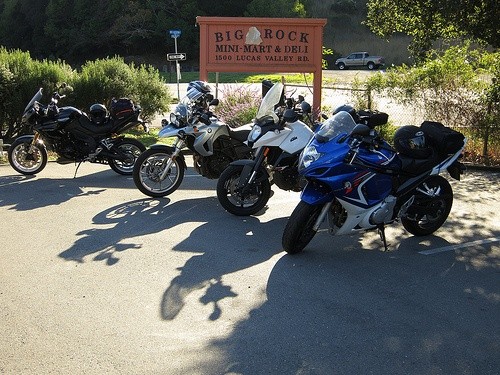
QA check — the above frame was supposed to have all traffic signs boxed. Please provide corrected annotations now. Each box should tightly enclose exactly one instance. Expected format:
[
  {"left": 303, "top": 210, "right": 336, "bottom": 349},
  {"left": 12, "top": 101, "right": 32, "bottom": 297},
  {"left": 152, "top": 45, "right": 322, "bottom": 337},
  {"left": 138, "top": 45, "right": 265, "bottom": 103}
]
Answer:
[{"left": 167, "top": 52, "right": 187, "bottom": 61}]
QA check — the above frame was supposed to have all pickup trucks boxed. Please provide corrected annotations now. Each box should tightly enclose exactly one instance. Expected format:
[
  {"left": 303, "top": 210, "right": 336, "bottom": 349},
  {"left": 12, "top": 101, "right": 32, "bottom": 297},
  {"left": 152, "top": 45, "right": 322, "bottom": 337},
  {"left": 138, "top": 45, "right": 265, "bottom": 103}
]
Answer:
[{"left": 335, "top": 51, "right": 384, "bottom": 70}]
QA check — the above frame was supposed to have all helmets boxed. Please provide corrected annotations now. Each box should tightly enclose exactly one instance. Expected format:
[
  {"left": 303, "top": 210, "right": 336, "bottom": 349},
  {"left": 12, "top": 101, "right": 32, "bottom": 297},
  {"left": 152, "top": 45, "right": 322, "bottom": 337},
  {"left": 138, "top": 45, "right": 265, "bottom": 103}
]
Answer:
[
  {"left": 395, "top": 125, "right": 428, "bottom": 158},
  {"left": 187, "top": 81, "right": 211, "bottom": 101},
  {"left": 90, "top": 104, "right": 107, "bottom": 120},
  {"left": 333, "top": 105, "right": 356, "bottom": 122}
]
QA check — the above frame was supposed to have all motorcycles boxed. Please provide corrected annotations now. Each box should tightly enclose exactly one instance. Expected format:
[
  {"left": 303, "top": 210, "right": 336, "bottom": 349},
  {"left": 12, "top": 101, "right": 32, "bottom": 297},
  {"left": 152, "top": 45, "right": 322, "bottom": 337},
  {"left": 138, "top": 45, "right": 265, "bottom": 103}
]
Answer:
[
  {"left": 8, "top": 82, "right": 149, "bottom": 175},
  {"left": 132, "top": 80, "right": 468, "bottom": 255}
]
